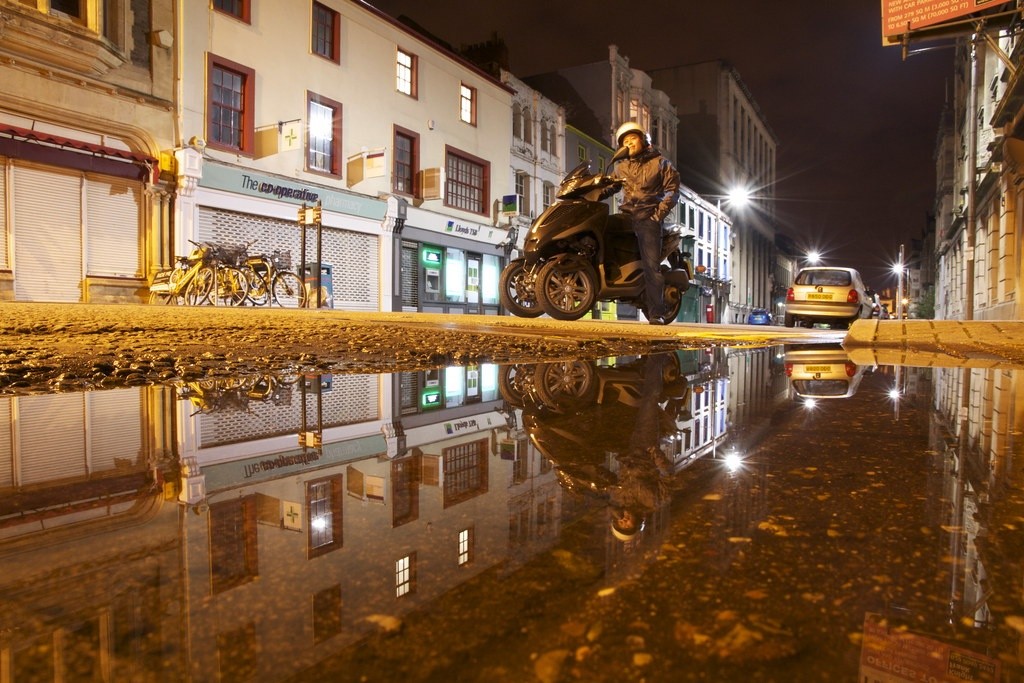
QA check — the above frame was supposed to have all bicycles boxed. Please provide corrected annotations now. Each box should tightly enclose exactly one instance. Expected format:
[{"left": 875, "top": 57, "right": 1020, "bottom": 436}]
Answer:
[{"left": 148, "top": 238, "right": 307, "bottom": 310}]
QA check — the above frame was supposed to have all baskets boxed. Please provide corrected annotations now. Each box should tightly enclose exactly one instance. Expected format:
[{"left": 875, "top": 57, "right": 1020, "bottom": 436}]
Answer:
[
  {"left": 229, "top": 249, "right": 248, "bottom": 266},
  {"left": 271, "top": 251, "right": 291, "bottom": 268}
]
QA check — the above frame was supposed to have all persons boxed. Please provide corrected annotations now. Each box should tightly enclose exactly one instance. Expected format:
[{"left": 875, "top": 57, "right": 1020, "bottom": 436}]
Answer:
[
  {"left": 578, "top": 443, "right": 680, "bottom": 549},
  {"left": 601, "top": 121, "right": 681, "bottom": 324}
]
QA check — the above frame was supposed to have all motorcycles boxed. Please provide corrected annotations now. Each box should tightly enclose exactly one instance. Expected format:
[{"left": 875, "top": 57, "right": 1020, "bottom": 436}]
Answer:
[
  {"left": 498, "top": 353, "right": 714, "bottom": 501},
  {"left": 500, "top": 146, "right": 706, "bottom": 327}
]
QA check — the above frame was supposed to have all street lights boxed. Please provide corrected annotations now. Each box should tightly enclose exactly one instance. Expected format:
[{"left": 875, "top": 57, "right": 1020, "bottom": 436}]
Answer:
[{"left": 893, "top": 245, "right": 905, "bottom": 319}]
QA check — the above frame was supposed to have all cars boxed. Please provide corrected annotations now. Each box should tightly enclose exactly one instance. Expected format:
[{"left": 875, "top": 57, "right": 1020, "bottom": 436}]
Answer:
[
  {"left": 785, "top": 267, "right": 890, "bottom": 330},
  {"left": 748, "top": 308, "right": 772, "bottom": 325},
  {"left": 783, "top": 343, "right": 871, "bottom": 400}
]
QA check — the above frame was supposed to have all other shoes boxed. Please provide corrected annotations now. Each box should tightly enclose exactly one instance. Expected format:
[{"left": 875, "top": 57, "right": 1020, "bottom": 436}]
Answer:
[
  {"left": 649, "top": 309, "right": 665, "bottom": 325},
  {"left": 649, "top": 353, "right": 665, "bottom": 363},
  {"left": 606, "top": 272, "right": 622, "bottom": 283}
]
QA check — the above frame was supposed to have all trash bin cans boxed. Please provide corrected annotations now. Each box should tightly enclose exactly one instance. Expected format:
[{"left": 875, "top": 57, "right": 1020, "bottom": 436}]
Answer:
[
  {"left": 706, "top": 304, "right": 713, "bottom": 323},
  {"left": 296, "top": 262, "right": 334, "bottom": 309}
]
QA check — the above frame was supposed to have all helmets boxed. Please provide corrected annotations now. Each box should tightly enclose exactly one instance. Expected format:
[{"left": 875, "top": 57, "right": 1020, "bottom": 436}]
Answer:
[
  {"left": 616, "top": 122, "right": 651, "bottom": 146},
  {"left": 611, "top": 520, "right": 645, "bottom": 541}
]
